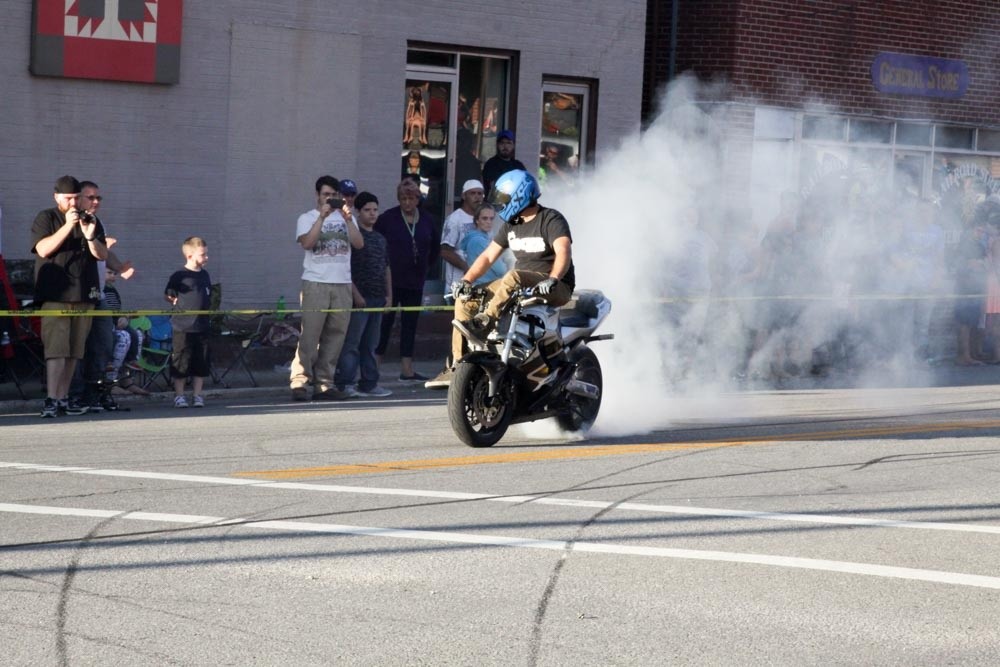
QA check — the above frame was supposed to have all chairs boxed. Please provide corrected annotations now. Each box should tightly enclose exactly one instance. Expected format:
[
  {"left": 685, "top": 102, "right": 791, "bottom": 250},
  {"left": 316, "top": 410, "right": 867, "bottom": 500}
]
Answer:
[
  {"left": 212, "top": 311, "right": 272, "bottom": 388},
  {"left": 125, "top": 335, "right": 176, "bottom": 394}
]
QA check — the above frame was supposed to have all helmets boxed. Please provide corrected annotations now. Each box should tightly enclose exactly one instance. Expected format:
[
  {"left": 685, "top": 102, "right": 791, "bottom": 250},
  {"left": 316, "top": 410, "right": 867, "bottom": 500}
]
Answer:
[{"left": 493, "top": 169, "right": 542, "bottom": 224}]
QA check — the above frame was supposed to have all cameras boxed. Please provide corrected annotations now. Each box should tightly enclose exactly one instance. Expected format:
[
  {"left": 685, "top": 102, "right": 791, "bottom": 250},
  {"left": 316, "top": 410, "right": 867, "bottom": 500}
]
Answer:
[{"left": 75, "top": 209, "right": 93, "bottom": 224}]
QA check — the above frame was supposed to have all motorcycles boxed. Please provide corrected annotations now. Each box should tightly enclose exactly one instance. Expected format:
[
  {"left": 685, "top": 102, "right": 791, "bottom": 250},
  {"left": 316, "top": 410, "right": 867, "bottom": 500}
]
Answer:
[{"left": 443, "top": 288, "right": 614, "bottom": 447}]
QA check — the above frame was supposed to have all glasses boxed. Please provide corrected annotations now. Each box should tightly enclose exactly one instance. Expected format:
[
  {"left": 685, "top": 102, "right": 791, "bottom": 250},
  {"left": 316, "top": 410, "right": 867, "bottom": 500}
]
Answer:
[{"left": 80, "top": 196, "right": 102, "bottom": 201}]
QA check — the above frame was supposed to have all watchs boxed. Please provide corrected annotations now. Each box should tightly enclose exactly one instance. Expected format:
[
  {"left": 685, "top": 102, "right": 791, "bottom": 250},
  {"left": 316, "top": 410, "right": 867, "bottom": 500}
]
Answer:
[{"left": 344, "top": 218, "right": 352, "bottom": 223}]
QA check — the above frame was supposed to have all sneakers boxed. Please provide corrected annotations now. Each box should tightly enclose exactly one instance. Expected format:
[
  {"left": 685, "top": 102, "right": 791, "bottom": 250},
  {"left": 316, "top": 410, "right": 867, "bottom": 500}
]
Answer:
[
  {"left": 424, "top": 367, "right": 456, "bottom": 390},
  {"left": 58, "top": 399, "right": 90, "bottom": 417},
  {"left": 332, "top": 384, "right": 360, "bottom": 397},
  {"left": 312, "top": 389, "right": 348, "bottom": 400},
  {"left": 41, "top": 398, "right": 58, "bottom": 419},
  {"left": 451, "top": 318, "right": 490, "bottom": 350},
  {"left": 293, "top": 388, "right": 307, "bottom": 400},
  {"left": 174, "top": 394, "right": 189, "bottom": 407},
  {"left": 356, "top": 384, "right": 392, "bottom": 397},
  {"left": 192, "top": 394, "right": 204, "bottom": 407}
]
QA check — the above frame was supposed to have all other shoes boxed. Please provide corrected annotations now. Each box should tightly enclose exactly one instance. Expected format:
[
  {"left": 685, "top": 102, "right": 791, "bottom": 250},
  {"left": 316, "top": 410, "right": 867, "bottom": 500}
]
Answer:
[
  {"left": 78, "top": 398, "right": 104, "bottom": 412},
  {"left": 398, "top": 372, "right": 429, "bottom": 383},
  {"left": 125, "top": 359, "right": 143, "bottom": 371},
  {"left": 122, "top": 383, "right": 149, "bottom": 395},
  {"left": 111, "top": 385, "right": 133, "bottom": 395},
  {"left": 101, "top": 400, "right": 119, "bottom": 410},
  {"left": 105, "top": 361, "right": 114, "bottom": 372}
]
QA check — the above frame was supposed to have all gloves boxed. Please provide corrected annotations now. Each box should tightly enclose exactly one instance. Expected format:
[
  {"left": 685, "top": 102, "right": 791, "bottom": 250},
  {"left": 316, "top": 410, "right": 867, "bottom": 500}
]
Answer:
[
  {"left": 451, "top": 280, "right": 472, "bottom": 299},
  {"left": 530, "top": 277, "right": 558, "bottom": 296}
]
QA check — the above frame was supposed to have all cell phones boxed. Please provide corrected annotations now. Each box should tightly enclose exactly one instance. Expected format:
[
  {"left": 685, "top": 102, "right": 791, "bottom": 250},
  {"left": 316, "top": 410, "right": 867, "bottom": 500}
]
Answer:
[{"left": 326, "top": 199, "right": 347, "bottom": 209}]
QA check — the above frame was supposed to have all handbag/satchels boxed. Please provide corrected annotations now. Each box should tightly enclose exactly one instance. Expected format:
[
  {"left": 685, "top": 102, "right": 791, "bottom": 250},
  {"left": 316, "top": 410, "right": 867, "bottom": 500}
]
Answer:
[{"left": 261, "top": 321, "right": 300, "bottom": 349}]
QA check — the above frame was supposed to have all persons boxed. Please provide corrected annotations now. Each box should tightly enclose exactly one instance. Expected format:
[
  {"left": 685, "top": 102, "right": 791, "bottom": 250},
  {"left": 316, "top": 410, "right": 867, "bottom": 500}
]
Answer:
[
  {"left": 540, "top": 145, "right": 578, "bottom": 183},
  {"left": 482, "top": 130, "right": 527, "bottom": 200},
  {"left": 425, "top": 169, "right": 575, "bottom": 389},
  {"left": 440, "top": 180, "right": 507, "bottom": 306},
  {"left": 672, "top": 194, "right": 1000, "bottom": 390},
  {"left": 164, "top": 237, "right": 211, "bottom": 408},
  {"left": 31, "top": 175, "right": 143, "bottom": 418},
  {"left": 290, "top": 175, "right": 434, "bottom": 400}
]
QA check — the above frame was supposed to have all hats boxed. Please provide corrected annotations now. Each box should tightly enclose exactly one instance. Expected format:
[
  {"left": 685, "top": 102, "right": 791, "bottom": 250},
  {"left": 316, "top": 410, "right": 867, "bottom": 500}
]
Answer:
[
  {"left": 338, "top": 180, "right": 358, "bottom": 195},
  {"left": 54, "top": 176, "right": 81, "bottom": 193},
  {"left": 462, "top": 180, "right": 484, "bottom": 193},
  {"left": 497, "top": 130, "right": 514, "bottom": 141}
]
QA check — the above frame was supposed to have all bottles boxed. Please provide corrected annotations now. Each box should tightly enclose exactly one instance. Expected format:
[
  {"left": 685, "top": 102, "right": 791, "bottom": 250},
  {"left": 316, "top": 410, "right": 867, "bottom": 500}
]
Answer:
[
  {"left": 424, "top": 294, "right": 431, "bottom": 312},
  {"left": 2, "top": 332, "right": 9, "bottom": 344},
  {"left": 277, "top": 296, "right": 287, "bottom": 319}
]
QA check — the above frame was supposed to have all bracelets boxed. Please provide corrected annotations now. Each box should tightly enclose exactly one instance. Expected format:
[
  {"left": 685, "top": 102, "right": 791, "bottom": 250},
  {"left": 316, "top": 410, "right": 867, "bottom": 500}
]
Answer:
[{"left": 86, "top": 236, "right": 97, "bottom": 241}]
